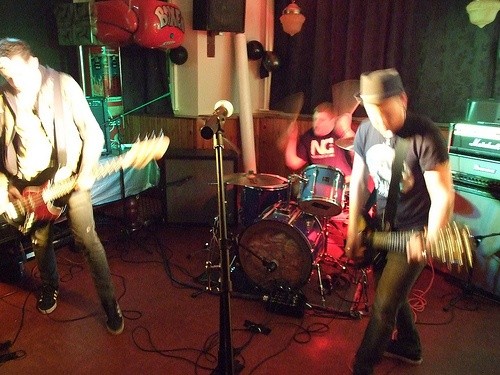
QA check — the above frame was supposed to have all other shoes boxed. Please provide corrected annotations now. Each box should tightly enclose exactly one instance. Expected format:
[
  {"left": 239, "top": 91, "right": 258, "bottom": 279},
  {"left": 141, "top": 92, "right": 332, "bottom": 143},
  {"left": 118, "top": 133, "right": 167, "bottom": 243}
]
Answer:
[{"left": 384, "top": 339, "right": 422, "bottom": 364}]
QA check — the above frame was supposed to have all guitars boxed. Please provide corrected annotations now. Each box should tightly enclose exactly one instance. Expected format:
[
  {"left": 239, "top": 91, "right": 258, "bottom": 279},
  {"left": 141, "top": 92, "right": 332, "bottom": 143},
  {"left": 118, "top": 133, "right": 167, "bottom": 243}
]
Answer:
[
  {"left": 347, "top": 211, "right": 479, "bottom": 276},
  {"left": 1, "top": 128, "right": 171, "bottom": 235}
]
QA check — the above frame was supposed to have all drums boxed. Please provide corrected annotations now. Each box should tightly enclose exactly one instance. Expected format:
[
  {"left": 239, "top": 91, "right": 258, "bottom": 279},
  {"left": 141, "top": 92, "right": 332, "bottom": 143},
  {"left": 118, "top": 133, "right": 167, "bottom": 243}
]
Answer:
[
  {"left": 236, "top": 200, "right": 324, "bottom": 292},
  {"left": 242, "top": 184, "right": 292, "bottom": 225},
  {"left": 314, "top": 215, "right": 330, "bottom": 253},
  {"left": 297, "top": 163, "right": 345, "bottom": 217}
]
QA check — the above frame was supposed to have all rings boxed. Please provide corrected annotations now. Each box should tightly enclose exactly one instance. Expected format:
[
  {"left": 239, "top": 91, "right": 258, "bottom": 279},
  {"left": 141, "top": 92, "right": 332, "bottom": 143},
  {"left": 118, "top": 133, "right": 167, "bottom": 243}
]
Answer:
[{"left": 347, "top": 247, "right": 354, "bottom": 251}]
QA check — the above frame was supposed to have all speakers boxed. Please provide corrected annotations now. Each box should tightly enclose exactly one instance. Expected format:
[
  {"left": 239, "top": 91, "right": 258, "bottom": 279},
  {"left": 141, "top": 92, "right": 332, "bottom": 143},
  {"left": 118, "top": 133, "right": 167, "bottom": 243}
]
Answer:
[
  {"left": 425, "top": 182, "right": 500, "bottom": 302},
  {"left": 192, "top": 0, "right": 246, "bottom": 33}
]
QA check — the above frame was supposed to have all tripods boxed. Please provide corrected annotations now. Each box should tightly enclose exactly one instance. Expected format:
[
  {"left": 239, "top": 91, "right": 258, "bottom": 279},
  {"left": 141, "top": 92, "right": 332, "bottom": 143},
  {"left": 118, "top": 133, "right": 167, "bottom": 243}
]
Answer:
[{"left": 97, "top": 169, "right": 153, "bottom": 254}]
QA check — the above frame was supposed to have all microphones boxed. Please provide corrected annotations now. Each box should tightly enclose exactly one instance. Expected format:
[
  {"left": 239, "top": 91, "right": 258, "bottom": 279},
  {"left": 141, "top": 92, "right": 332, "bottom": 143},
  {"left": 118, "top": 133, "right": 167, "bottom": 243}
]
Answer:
[{"left": 201, "top": 100, "right": 233, "bottom": 140}]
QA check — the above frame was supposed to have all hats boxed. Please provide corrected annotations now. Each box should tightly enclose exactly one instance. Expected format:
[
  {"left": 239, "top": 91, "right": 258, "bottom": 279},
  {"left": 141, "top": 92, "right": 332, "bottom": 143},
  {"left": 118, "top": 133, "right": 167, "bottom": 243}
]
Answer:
[{"left": 354, "top": 68, "right": 403, "bottom": 100}]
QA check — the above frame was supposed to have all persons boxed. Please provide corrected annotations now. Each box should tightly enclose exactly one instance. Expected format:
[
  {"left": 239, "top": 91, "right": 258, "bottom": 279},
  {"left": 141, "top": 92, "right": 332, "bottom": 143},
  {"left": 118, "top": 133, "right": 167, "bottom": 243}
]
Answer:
[
  {"left": 346, "top": 67, "right": 455, "bottom": 375},
  {"left": 284, "top": 102, "right": 354, "bottom": 176},
  {"left": 0, "top": 37, "right": 124, "bottom": 334}
]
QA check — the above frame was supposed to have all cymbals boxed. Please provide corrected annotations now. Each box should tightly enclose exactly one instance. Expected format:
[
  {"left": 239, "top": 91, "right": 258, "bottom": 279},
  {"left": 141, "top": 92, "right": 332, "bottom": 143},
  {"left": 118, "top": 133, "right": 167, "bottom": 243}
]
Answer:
[
  {"left": 335, "top": 136, "right": 355, "bottom": 153},
  {"left": 224, "top": 173, "right": 290, "bottom": 187}
]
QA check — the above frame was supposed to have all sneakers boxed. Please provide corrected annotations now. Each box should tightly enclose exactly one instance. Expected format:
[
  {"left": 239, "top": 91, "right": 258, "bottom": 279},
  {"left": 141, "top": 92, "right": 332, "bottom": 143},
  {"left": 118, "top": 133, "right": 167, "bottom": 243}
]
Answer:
[
  {"left": 105, "top": 302, "right": 124, "bottom": 335},
  {"left": 38, "top": 286, "right": 59, "bottom": 315}
]
